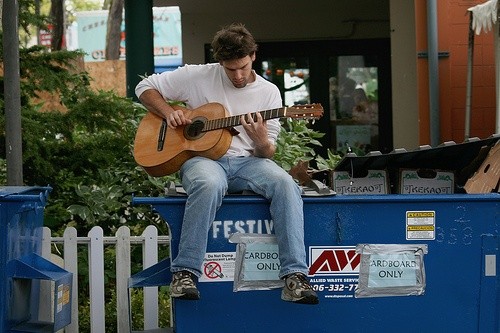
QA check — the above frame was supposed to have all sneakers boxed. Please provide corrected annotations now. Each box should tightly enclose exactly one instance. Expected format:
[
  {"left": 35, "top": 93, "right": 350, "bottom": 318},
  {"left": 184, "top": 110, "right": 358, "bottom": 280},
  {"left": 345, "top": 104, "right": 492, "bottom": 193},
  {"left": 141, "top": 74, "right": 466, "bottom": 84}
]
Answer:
[
  {"left": 281, "top": 272, "right": 319, "bottom": 305},
  {"left": 169, "top": 272, "right": 201, "bottom": 300}
]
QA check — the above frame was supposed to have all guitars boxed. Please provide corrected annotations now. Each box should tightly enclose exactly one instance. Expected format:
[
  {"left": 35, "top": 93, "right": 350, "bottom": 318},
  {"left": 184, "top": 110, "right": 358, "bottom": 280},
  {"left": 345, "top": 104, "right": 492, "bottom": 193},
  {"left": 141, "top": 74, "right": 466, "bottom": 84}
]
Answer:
[{"left": 132, "top": 101, "right": 325, "bottom": 179}]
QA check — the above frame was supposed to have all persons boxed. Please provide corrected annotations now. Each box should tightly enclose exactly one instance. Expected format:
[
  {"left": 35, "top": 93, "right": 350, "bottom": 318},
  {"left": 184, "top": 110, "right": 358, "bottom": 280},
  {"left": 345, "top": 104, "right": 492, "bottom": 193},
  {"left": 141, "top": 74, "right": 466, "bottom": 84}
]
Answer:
[{"left": 135, "top": 22, "right": 320, "bottom": 305}]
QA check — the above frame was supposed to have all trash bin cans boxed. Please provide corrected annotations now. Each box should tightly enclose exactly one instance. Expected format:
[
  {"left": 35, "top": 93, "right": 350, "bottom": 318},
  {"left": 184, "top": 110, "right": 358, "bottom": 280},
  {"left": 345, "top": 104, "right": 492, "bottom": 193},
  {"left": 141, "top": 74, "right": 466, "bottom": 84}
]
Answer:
[{"left": 1, "top": 185, "right": 74, "bottom": 333}]
